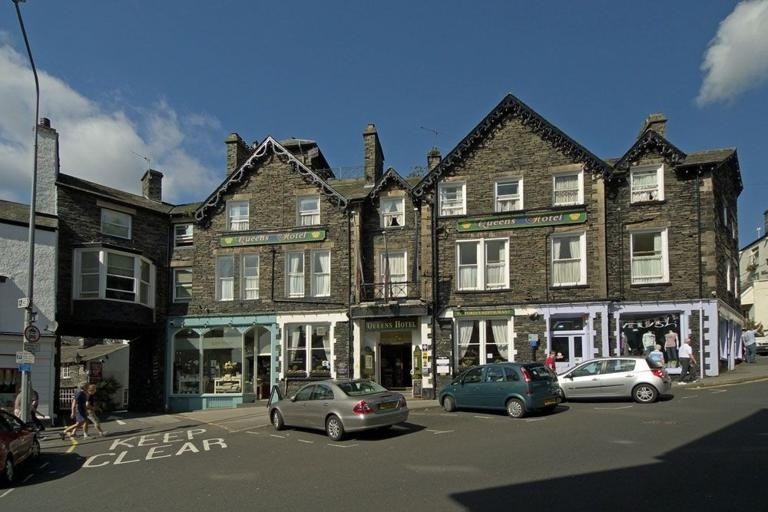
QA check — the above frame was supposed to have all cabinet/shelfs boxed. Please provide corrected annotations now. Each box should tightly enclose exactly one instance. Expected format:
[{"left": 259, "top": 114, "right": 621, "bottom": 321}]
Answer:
[{"left": 178, "top": 377, "right": 241, "bottom": 393}]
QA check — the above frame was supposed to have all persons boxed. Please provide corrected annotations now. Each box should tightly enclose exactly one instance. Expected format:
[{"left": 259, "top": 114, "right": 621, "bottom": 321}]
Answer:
[
  {"left": 542, "top": 351, "right": 556, "bottom": 373},
  {"left": 615, "top": 331, "right": 631, "bottom": 356},
  {"left": 646, "top": 344, "right": 664, "bottom": 373},
  {"left": 13, "top": 391, "right": 22, "bottom": 440},
  {"left": 641, "top": 329, "right": 656, "bottom": 357},
  {"left": 70, "top": 384, "right": 109, "bottom": 441},
  {"left": 676, "top": 337, "right": 696, "bottom": 387},
  {"left": 30, "top": 390, "right": 45, "bottom": 436},
  {"left": 742, "top": 321, "right": 763, "bottom": 364},
  {"left": 57, "top": 382, "right": 89, "bottom": 441},
  {"left": 663, "top": 329, "right": 679, "bottom": 363}
]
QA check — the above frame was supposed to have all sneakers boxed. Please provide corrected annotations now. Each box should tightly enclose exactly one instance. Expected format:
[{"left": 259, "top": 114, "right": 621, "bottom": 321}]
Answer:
[
  {"left": 59, "top": 432, "right": 66, "bottom": 440},
  {"left": 71, "top": 430, "right": 109, "bottom": 440}
]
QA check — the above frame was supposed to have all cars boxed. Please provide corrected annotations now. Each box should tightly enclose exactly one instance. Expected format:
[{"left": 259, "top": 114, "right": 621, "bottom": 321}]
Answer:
[
  {"left": 0, "top": 409, "right": 40, "bottom": 481},
  {"left": 269, "top": 380, "right": 409, "bottom": 441},
  {"left": 439, "top": 362, "right": 562, "bottom": 418},
  {"left": 556, "top": 358, "right": 670, "bottom": 403}
]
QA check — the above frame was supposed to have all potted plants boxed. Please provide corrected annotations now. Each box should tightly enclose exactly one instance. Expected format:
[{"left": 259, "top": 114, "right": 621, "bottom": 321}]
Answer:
[{"left": 223, "top": 360, "right": 238, "bottom": 377}]
